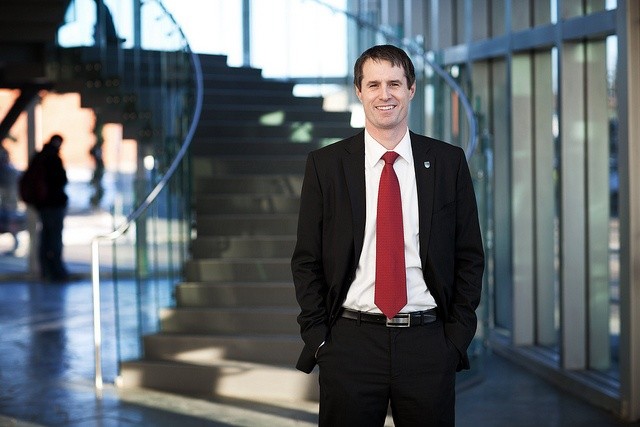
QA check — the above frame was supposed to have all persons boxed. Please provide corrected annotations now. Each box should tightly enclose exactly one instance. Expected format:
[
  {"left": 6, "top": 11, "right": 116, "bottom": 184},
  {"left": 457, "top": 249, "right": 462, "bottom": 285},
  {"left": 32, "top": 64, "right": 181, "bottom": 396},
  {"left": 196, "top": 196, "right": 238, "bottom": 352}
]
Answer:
[
  {"left": 288, "top": 46, "right": 487, "bottom": 426},
  {"left": 21, "top": 134, "right": 81, "bottom": 284}
]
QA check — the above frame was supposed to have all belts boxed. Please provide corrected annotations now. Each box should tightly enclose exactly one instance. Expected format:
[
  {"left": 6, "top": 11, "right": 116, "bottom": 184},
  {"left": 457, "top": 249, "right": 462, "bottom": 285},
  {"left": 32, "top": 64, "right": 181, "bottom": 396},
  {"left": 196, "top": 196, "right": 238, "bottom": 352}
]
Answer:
[{"left": 341, "top": 308, "right": 437, "bottom": 327}]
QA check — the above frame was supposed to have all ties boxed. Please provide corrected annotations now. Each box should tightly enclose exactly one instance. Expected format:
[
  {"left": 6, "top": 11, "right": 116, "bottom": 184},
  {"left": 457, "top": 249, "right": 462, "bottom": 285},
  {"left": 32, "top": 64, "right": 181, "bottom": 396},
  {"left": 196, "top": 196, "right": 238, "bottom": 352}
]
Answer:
[{"left": 375, "top": 152, "right": 407, "bottom": 320}]
